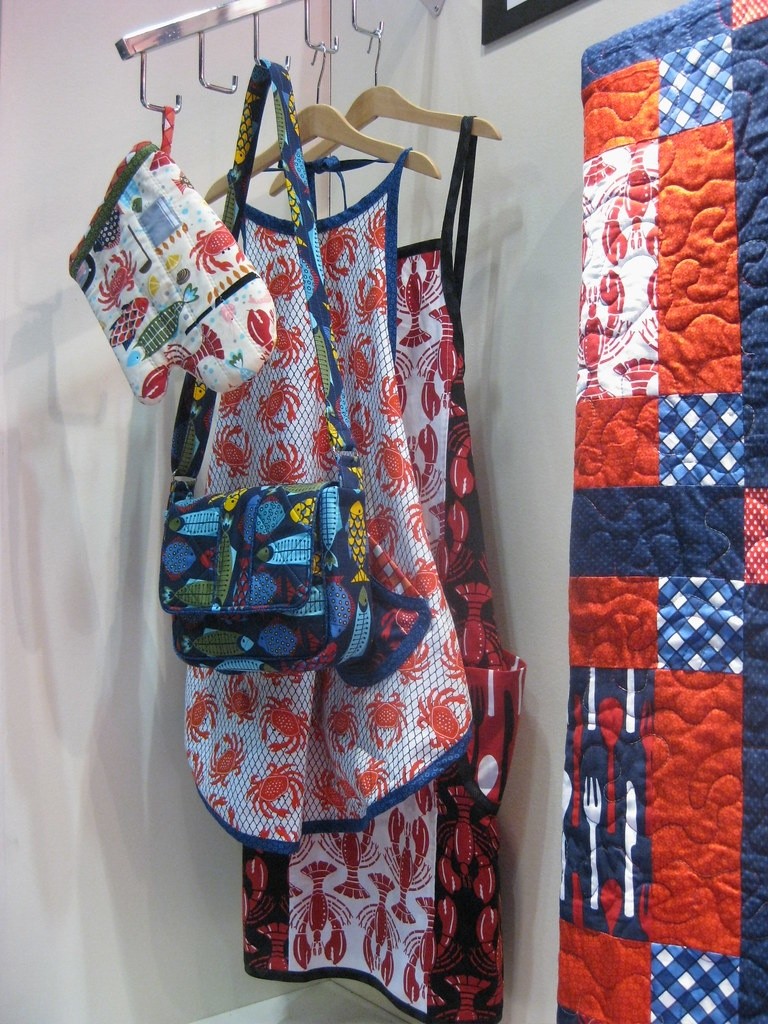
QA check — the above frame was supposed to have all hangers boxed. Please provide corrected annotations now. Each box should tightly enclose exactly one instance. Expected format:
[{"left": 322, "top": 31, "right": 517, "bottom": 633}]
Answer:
[
  {"left": 270, "top": 30, "right": 502, "bottom": 197},
  {"left": 204, "top": 41, "right": 441, "bottom": 206}
]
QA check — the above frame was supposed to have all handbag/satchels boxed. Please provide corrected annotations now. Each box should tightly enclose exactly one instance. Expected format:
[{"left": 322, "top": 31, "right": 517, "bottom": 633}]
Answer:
[
  {"left": 157, "top": 58, "right": 375, "bottom": 673},
  {"left": 68, "top": 105, "right": 277, "bottom": 406}
]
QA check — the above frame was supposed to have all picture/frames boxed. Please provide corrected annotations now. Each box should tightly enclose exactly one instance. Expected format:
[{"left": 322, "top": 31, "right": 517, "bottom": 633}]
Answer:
[{"left": 481, "top": 0, "right": 577, "bottom": 45}]
